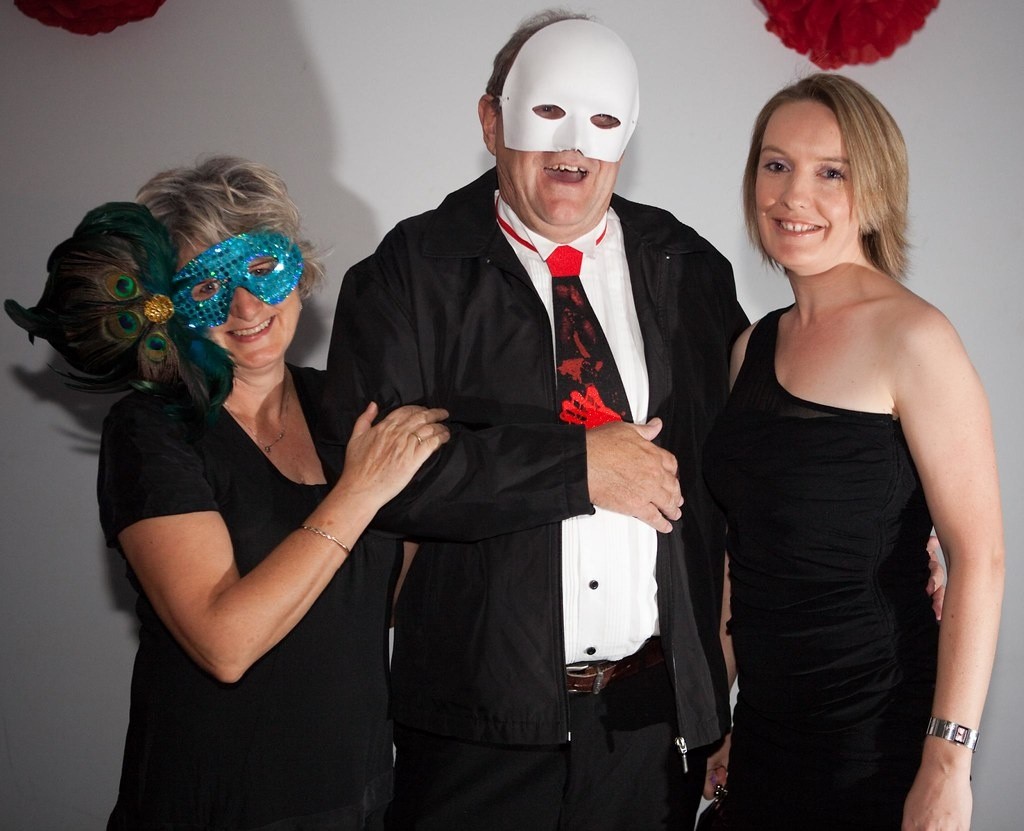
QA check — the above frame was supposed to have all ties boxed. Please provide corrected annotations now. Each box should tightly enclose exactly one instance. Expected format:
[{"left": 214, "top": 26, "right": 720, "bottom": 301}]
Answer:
[{"left": 541, "top": 245, "right": 633, "bottom": 432}]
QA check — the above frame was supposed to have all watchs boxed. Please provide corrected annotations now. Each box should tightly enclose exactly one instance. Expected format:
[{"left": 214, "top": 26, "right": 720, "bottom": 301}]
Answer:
[{"left": 923, "top": 715, "right": 981, "bottom": 754}]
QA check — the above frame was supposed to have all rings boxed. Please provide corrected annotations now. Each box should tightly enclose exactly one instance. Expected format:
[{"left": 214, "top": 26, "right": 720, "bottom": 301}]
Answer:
[{"left": 410, "top": 432, "right": 423, "bottom": 446}]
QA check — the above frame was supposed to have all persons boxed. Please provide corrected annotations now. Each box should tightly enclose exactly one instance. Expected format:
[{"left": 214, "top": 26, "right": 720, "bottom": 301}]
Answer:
[
  {"left": 319, "top": 5, "right": 951, "bottom": 830},
  {"left": 694, "top": 69, "right": 1008, "bottom": 831},
  {"left": 93, "top": 141, "right": 455, "bottom": 831}
]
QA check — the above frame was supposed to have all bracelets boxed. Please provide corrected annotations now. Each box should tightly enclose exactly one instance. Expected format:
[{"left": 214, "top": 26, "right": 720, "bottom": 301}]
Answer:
[{"left": 296, "top": 524, "right": 352, "bottom": 557}]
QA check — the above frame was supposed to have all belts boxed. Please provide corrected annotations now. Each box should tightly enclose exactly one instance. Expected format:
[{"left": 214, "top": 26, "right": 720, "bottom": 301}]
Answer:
[{"left": 562, "top": 649, "right": 669, "bottom": 695}]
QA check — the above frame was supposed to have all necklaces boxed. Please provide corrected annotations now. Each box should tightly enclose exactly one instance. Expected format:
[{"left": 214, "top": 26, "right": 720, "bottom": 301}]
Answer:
[{"left": 222, "top": 370, "right": 294, "bottom": 454}]
